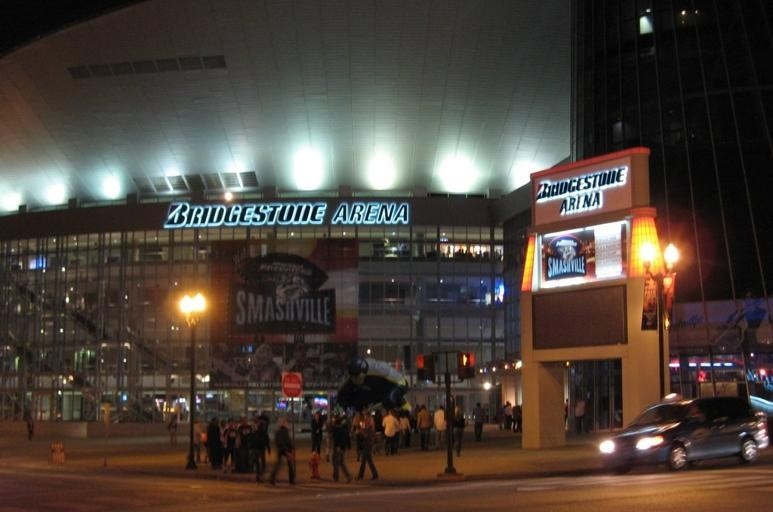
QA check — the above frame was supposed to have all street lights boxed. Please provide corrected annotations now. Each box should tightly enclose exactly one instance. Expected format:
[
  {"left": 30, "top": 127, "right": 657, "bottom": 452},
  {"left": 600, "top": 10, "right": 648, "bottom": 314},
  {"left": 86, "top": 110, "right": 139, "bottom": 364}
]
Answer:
[
  {"left": 638, "top": 241, "right": 679, "bottom": 402},
  {"left": 178, "top": 293, "right": 212, "bottom": 472}
]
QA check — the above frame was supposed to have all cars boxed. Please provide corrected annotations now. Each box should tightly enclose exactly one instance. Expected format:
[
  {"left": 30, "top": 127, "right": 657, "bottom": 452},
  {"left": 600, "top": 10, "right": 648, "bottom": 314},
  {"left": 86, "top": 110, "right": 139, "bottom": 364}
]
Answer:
[{"left": 596, "top": 396, "right": 771, "bottom": 473}]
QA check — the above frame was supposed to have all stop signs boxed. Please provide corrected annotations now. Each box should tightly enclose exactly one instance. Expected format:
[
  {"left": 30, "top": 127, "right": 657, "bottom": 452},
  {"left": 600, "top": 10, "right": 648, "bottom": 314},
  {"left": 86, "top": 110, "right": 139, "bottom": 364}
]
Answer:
[{"left": 281, "top": 372, "right": 304, "bottom": 398}]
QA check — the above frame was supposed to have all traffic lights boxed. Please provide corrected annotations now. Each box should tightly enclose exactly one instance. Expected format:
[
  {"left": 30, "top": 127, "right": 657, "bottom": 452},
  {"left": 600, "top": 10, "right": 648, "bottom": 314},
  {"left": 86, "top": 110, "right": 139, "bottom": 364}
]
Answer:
[
  {"left": 416, "top": 354, "right": 434, "bottom": 382},
  {"left": 458, "top": 352, "right": 476, "bottom": 379}
]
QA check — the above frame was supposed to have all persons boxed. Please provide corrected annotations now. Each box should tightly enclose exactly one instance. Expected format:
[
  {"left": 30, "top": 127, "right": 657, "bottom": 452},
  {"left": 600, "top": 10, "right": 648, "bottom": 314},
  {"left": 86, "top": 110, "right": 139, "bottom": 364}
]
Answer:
[
  {"left": 575, "top": 398, "right": 587, "bottom": 433},
  {"left": 166, "top": 415, "right": 179, "bottom": 447},
  {"left": 25, "top": 414, "right": 35, "bottom": 440},
  {"left": 190, "top": 399, "right": 524, "bottom": 486},
  {"left": 563, "top": 398, "right": 570, "bottom": 425}
]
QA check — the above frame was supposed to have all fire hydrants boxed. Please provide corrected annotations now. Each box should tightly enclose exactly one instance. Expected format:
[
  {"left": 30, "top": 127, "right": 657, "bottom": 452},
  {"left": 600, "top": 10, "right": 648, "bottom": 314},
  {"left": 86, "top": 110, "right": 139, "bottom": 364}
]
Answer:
[{"left": 308, "top": 451, "right": 322, "bottom": 479}]
